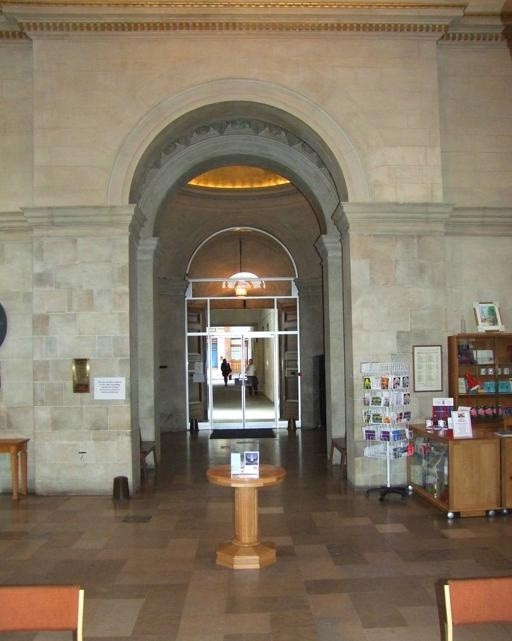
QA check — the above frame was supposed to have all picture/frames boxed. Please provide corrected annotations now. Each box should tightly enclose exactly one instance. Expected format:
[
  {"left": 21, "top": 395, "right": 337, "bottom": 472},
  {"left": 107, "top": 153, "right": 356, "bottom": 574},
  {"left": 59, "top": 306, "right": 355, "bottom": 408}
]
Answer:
[{"left": 472, "top": 299, "right": 507, "bottom": 334}]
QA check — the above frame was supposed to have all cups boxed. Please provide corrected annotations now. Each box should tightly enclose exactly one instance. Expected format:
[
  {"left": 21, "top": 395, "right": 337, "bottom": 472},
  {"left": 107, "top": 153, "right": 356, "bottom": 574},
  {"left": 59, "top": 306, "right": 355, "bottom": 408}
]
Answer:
[
  {"left": 426, "top": 420, "right": 433, "bottom": 427},
  {"left": 438, "top": 420, "right": 446, "bottom": 428},
  {"left": 480, "top": 368, "right": 509, "bottom": 375}
]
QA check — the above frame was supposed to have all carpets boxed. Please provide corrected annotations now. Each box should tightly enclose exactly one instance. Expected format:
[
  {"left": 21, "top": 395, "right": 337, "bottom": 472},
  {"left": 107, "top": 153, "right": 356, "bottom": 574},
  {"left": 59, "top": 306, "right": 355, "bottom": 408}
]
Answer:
[{"left": 210, "top": 428, "right": 276, "bottom": 439}]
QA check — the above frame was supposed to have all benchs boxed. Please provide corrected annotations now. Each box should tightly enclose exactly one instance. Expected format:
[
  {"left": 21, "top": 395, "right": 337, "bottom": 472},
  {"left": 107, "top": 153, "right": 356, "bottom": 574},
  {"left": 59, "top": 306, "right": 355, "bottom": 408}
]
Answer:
[
  {"left": 139, "top": 424, "right": 158, "bottom": 481},
  {"left": 330, "top": 437, "right": 347, "bottom": 471}
]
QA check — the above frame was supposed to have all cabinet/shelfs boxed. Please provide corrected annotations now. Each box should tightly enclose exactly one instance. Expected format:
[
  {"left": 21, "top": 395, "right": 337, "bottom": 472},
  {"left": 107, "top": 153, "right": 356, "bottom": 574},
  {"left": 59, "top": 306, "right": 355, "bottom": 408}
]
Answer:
[{"left": 405, "top": 333, "right": 512, "bottom": 520}]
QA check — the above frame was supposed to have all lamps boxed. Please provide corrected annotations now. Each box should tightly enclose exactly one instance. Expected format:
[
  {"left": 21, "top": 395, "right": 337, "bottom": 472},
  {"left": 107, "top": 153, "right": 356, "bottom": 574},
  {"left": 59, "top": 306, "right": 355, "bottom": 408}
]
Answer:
[{"left": 233, "top": 234, "right": 248, "bottom": 298}]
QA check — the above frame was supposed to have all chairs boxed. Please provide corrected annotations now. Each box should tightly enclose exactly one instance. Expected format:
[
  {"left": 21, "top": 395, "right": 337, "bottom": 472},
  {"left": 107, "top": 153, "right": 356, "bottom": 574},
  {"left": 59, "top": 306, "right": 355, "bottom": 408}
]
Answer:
[
  {"left": 0, "top": 586, "right": 84, "bottom": 641},
  {"left": 0, "top": 437, "right": 30, "bottom": 502},
  {"left": 438, "top": 578, "right": 511, "bottom": 640}
]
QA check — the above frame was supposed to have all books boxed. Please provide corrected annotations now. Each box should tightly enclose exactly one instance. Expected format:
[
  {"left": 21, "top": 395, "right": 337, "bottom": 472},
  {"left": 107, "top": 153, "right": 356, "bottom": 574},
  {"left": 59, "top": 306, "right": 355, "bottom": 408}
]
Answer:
[
  {"left": 362, "top": 373, "right": 415, "bottom": 458},
  {"left": 494, "top": 430, "right": 511, "bottom": 438}
]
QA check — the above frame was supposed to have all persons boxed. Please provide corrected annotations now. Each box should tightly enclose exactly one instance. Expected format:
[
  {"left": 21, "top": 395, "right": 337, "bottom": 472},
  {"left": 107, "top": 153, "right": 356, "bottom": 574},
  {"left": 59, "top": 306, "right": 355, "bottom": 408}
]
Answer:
[
  {"left": 220, "top": 359, "right": 232, "bottom": 388},
  {"left": 245, "top": 359, "right": 260, "bottom": 399}
]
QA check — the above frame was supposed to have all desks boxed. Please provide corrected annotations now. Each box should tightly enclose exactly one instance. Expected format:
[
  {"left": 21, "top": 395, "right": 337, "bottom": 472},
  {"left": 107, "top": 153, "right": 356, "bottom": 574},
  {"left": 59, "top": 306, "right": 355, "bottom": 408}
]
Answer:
[{"left": 205, "top": 463, "right": 285, "bottom": 571}]
